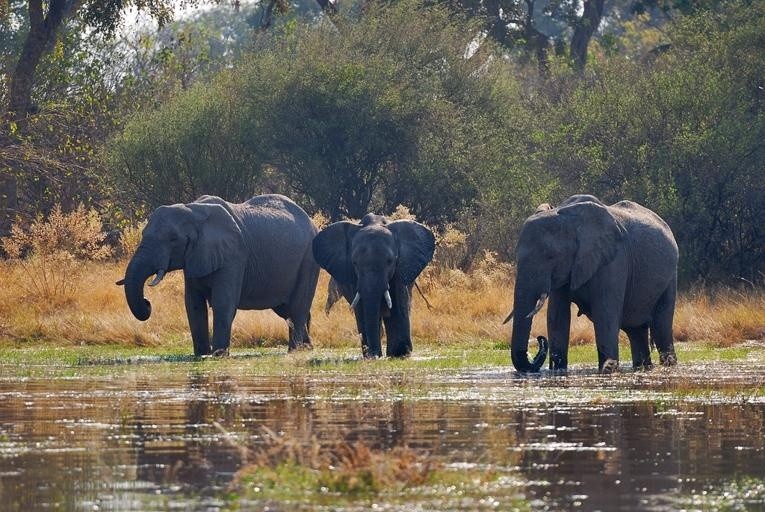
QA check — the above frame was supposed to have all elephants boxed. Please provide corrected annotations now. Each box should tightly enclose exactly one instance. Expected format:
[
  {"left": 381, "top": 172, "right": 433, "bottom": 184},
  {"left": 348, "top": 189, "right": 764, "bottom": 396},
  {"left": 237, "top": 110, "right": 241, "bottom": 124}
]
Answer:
[
  {"left": 502, "top": 193, "right": 679, "bottom": 374},
  {"left": 312, "top": 212, "right": 436, "bottom": 359},
  {"left": 116, "top": 194, "right": 322, "bottom": 358}
]
